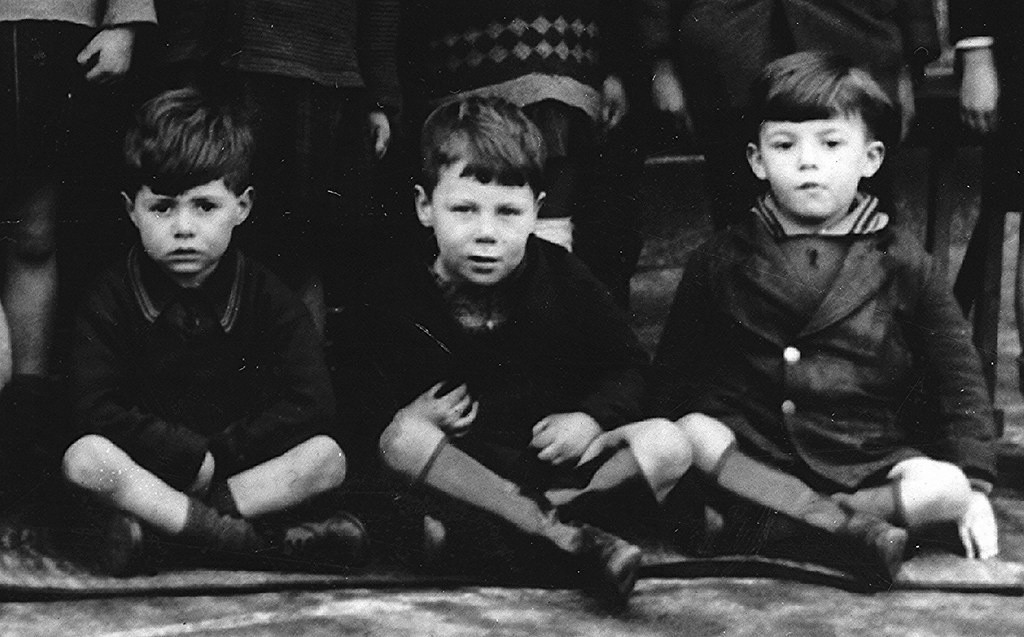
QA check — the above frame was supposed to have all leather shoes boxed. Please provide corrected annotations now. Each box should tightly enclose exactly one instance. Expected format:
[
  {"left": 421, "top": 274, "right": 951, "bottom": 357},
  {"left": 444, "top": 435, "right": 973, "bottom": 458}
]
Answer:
[
  {"left": 254, "top": 513, "right": 371, "bottom": 574},
  {"left": 836, "top": 512, "right": 908, "bottom": 579},
  {"left": 670, "top": 502, "right": 750, "bottom": 556},
  {"left": 366, "top": 495, "right": 451, "bottom": 566},
  {"left": 578, "top": 525, "right": 643, "bottom": 607},
  {"left": 102, "top": 512, "right": 153, "bottom": 577}
]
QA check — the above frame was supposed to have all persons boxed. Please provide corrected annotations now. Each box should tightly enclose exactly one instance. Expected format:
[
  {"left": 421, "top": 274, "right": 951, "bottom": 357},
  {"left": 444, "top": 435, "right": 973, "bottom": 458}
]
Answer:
[
  {"left": 326, "top": 95, "right": 693, "bottom": 602},
  {"left": 38, "top": 85, "right": 369, "bottom": 577},
  {"left": 653, "top": 51, "right": 1000, "bottom": 581},
  {"left": 1, "top": 0, "right": 1023, "bottom": 440}
]
